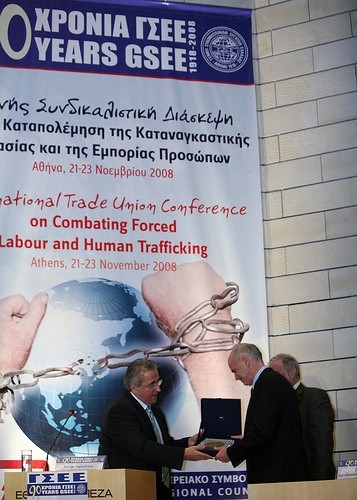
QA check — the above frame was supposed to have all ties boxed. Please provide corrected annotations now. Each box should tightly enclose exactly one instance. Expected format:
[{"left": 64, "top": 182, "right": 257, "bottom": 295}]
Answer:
[
  {"left": 145, "top": 406, "right": 170, "bottom": 489},
  {"left": 250, "top": 388, "right": 254, "bottom": 395}
]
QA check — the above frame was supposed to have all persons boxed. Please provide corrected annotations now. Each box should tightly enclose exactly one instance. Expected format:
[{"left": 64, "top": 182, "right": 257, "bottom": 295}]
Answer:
[
  {"left": 268, "top": 354, "right": 337, "bottom": 482},
  {"left": 0, "top": 260, "right": 252, "bottom": 437},
  {"left": 213, "top": 343, "right": 306, "bottom": 485},
  {"left": 98, "top": 358, "right": 214, "bottom": 500}
]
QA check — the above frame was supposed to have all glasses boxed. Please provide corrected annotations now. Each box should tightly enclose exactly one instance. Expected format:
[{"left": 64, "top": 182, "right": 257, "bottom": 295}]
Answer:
[{"left": 141, "top": 379, "right": 163, "bottom": 389}]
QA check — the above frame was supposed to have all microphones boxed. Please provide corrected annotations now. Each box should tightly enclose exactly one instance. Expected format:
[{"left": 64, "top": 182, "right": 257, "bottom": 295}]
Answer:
[{"left": 45, "top": 410, "right": 74, "bottom": 471}]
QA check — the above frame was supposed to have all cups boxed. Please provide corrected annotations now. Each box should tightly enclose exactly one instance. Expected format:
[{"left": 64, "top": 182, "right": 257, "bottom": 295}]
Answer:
[{"left": 22, "top": 450, "right": 32, "bottom": 472}]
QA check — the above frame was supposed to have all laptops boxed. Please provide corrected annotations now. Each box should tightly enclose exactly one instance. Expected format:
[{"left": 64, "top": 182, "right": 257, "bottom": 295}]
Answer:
[{"left": 192, "top": 397, "right": 242, "bottom": 445}]
